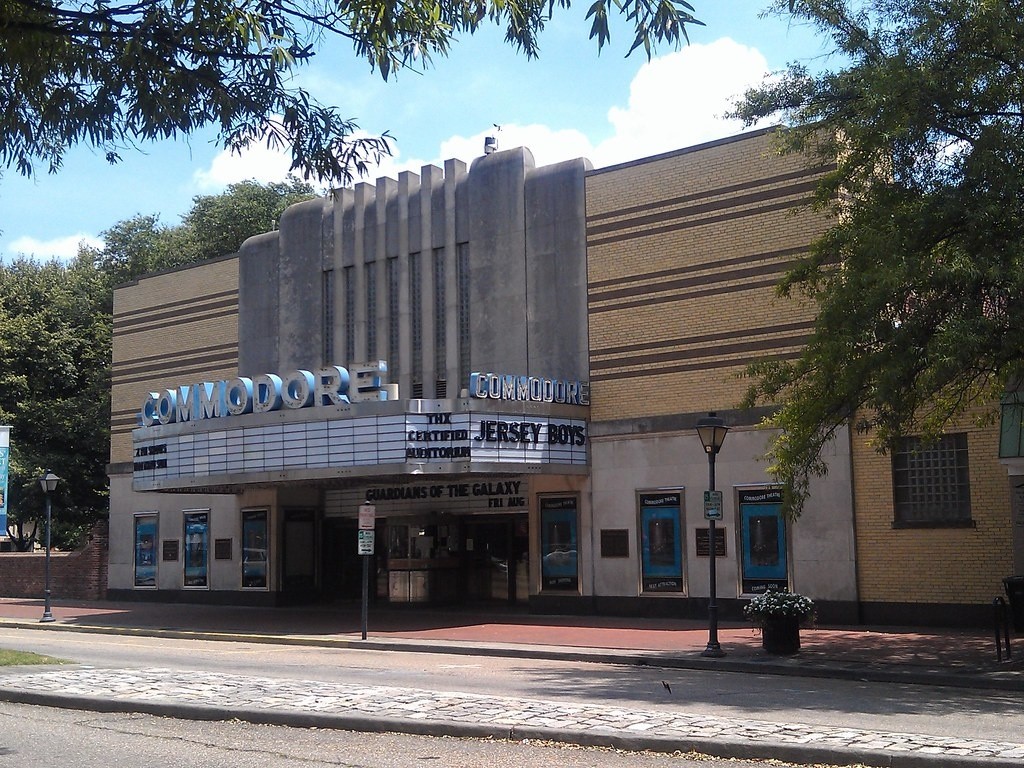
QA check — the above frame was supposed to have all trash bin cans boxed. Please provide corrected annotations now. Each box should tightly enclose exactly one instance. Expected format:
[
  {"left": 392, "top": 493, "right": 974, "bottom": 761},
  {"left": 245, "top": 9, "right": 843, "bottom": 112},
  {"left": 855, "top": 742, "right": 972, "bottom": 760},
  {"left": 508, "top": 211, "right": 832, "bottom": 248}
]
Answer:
[{"left": 1001, "top": 574, "right": 1024, "bottom": 634}]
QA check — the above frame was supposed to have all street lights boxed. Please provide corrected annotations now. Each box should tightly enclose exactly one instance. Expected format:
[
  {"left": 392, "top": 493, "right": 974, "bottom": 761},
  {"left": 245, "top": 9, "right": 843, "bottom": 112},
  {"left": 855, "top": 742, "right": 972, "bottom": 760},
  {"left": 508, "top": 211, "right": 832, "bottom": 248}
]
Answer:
[
  {"left": 695, "top": 408, "right": 735, "bottom": 654},
  {"left": 39, "top": 468, "right": 60, "bottom": 625}
]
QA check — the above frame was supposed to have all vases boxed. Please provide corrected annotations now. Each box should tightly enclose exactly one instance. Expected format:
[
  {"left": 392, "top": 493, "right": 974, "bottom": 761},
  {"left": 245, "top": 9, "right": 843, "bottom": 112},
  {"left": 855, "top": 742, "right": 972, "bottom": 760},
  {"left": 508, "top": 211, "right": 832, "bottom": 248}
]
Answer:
[{"left": 761, "top": 615, "right": 802, "bottom": 655}]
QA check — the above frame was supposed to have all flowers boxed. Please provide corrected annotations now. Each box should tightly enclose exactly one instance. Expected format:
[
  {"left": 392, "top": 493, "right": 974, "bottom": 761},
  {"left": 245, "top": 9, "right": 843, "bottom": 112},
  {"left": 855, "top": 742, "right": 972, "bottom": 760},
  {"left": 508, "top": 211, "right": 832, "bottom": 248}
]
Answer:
[{"left": 742, "top": 586, "right": 818, "bottom": 636}]
